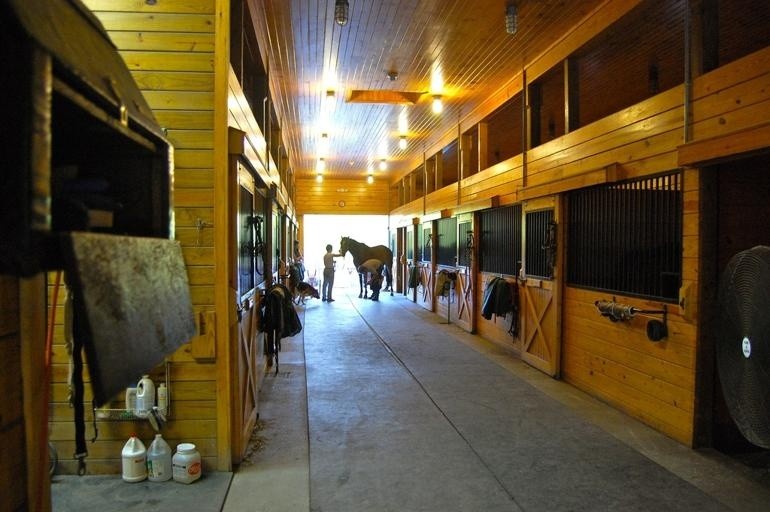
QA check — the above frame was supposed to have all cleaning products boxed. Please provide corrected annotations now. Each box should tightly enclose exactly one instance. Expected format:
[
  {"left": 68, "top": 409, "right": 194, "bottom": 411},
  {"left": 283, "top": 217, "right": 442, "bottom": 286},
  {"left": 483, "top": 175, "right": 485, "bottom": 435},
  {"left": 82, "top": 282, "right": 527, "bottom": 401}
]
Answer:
[{"left": 157, "top": 382, "right": 167, "bottom": 415}]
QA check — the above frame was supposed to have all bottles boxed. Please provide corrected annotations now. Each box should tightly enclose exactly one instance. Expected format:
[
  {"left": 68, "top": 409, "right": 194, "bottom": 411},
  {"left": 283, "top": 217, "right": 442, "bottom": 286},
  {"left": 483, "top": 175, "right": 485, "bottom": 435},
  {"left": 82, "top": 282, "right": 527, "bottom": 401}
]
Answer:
[{"left": 157, "top": 383, "right": 167, "bottom": 410}]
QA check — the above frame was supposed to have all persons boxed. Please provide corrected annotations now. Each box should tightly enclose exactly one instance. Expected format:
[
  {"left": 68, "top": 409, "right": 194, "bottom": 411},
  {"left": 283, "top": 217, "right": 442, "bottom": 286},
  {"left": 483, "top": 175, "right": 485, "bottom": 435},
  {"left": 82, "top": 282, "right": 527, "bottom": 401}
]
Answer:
[
  {"left": 294, "top": 240, "right": 302, "bottom": 264},
  {"left": 358, "top": 258, "right": 383, "bottom": 301},
  {"left": 322, "top": 244, "right": 341, "bottom": 302}
]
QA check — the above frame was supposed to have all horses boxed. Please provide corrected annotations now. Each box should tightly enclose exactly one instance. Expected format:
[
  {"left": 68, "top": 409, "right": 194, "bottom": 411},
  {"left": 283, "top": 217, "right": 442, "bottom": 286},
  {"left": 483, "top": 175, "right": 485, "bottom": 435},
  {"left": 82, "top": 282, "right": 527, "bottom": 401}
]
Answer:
[{"left": 339, "top": 235, "right": 394, "bottom": 298}]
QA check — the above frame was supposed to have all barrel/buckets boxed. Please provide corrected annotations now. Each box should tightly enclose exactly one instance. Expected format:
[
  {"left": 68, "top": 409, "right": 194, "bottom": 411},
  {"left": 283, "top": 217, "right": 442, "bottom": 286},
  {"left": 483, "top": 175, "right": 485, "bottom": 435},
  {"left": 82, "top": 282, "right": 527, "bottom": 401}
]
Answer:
[
  {"left": 120, "top": 431, "right": 148, "bottom": 482},
  {"left": 146, "top": 433, "right": 172, "bottom": 482},
  {"left": 135, "top": 374, "right": 155, "bottom": 419},
  {"left": 171, "top": 443, "right": 202, "bottom": 485}
]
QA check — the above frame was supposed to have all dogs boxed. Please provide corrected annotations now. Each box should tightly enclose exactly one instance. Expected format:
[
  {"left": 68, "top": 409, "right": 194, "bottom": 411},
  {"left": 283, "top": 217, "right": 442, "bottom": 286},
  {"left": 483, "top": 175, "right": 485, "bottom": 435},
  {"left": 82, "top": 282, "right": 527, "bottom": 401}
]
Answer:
[{"left": 291, "top": 281, "right": 320, "bottom": 306}]
